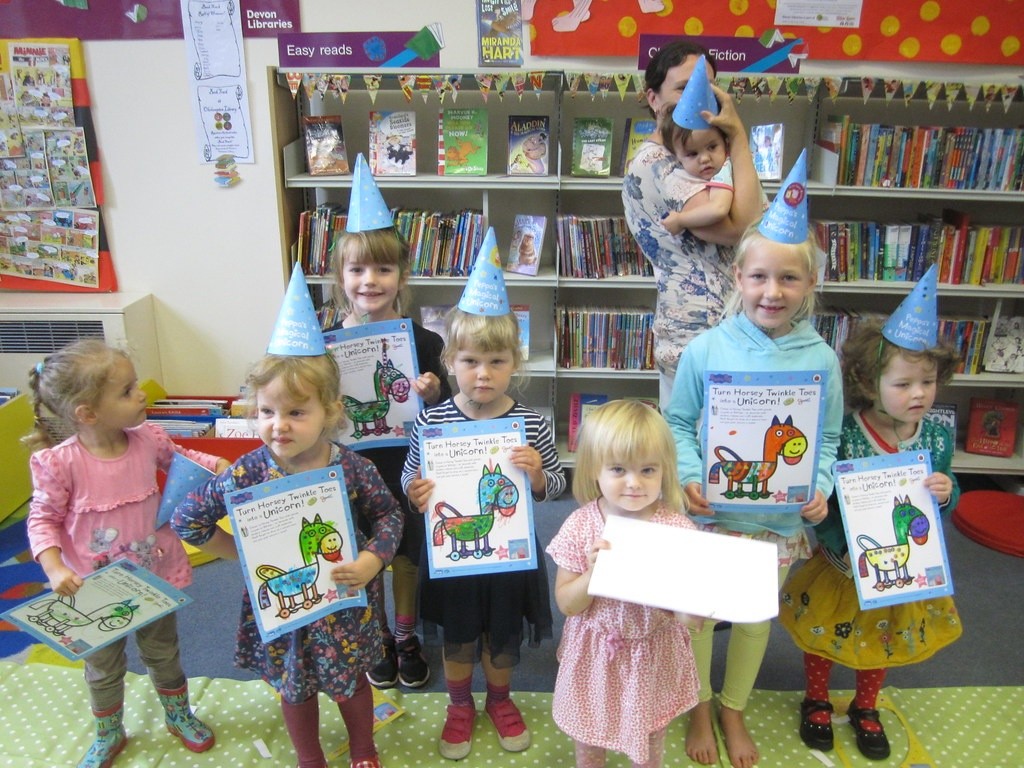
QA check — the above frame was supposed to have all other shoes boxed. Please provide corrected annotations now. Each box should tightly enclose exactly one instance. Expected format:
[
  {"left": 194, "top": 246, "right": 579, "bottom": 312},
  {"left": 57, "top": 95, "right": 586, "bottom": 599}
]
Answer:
[
  {"left": 847, "top": 704, "right": 890, "bottom": 760},
  {"left": 801, "top": 698, "right": 834, "bottom": 751}
]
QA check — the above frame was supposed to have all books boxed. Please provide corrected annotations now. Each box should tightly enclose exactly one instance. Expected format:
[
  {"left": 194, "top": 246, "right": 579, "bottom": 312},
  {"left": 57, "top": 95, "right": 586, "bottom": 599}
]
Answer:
[
  {"left": 289, "top": 114, "right": 1024, "bottom": 451},
  {"left": 142, "top": 396, "right": 266, "bottom": 440}
]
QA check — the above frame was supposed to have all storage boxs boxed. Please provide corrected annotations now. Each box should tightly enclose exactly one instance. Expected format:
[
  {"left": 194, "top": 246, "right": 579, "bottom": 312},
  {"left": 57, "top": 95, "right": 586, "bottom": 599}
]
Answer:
[{"left": 0, "top": 379, "right": 264, "bottom": 522}]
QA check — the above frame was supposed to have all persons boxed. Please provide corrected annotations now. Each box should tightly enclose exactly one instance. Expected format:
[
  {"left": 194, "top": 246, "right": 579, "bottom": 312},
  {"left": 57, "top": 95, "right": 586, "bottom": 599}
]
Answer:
[
  {"left": 659, "top": 101, "right": 772, "bottom": 236},
  {"left": 171, "top": 344, "right": 407, "bottom": 768},
  {"left": 544, "top": 396, "right": 706, "bottom": 767},
  {"left": 398, "top": 301, "right": 566, "bottom": 760},
  {"left": 24, "top": 340, "right": 231, "bottom": 768},
  {"left": 773, "top": 320, "right": 964, "bottom": 761},
  {"left": 318, "top": 226, "right": 452, "bottom": 688},
  {"left": 622, "top": 44, "right": 762, "bottom": 449},
  {"left": 664, "top": 215, "right": 845, "bottom": 767}
]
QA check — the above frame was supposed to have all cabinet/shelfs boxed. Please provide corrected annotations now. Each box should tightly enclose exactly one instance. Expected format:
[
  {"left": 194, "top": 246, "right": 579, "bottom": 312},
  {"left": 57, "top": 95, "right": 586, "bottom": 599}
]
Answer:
[{"left": 266, "top": 65, "right": 1024, "bottom": 475}]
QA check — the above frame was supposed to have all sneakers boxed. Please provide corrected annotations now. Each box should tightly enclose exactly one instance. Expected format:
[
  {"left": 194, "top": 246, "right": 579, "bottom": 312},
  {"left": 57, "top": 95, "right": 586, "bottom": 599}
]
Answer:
[
  {"left": 396, "top": 634, "right": 430, "bottom": 687},
  {"left": 484, "top": 698, "right": 530, "bottom": 751},
  {"left": 366, "top": 632, "right": 399, "bottom": 687},
  {"left": 439, "top": 704, "right": 477, "bottom": 759}
]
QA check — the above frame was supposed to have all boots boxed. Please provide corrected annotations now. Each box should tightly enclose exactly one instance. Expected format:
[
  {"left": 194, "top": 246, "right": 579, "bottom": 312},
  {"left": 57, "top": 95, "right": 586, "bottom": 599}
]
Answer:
[
  {"left": 156, "top": 678, "right": 214, "bottom": 752},
  {"left": 76, "top": 701, "right": 126, "bottom": 768}
]
